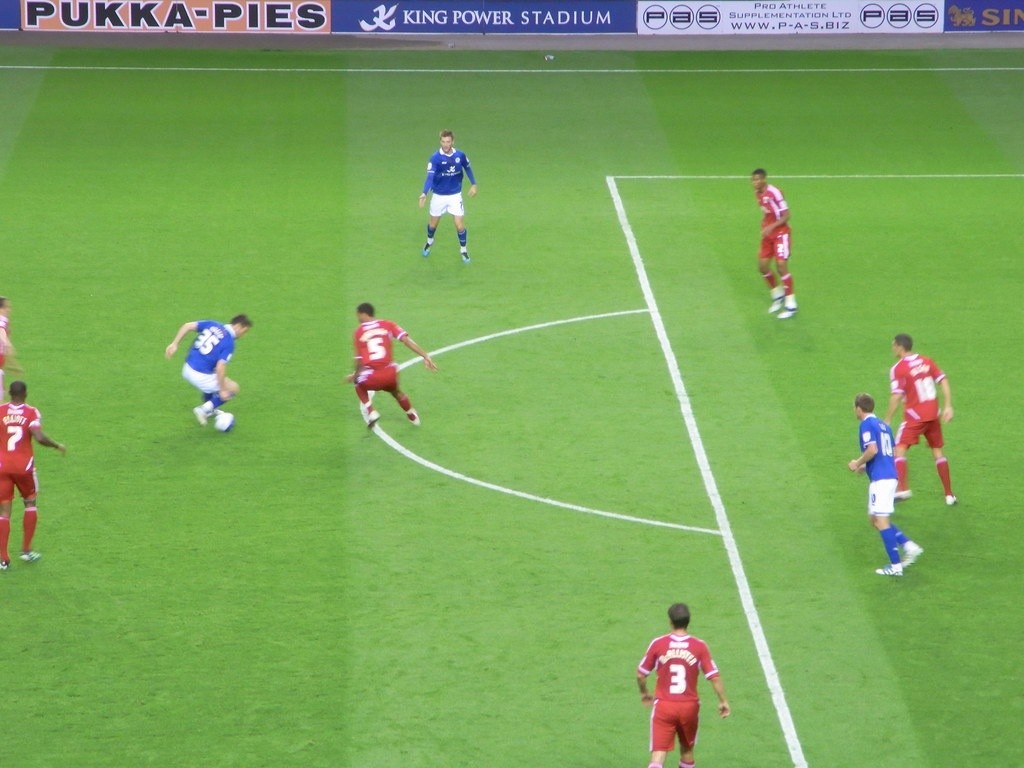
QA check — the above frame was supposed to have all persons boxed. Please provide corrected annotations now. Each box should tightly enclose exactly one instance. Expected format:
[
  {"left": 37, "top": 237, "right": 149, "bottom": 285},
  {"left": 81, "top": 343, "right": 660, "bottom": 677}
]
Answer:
[
  {"left": 751, "top": 169, "right": 798, "bottom": 320},
  {"left": 346, "top": 303, "right": 437, "bottom": 429},
  {"left": 166, "top": 315, "right": 252, "bottom": 433},
  {"left": 0, "top": 298, "right": 23, "bottom": 405},
  {"left": 637, "top": 602, "right": 731, "bottom": 768},
  {"left": 847, "top": 393, "right": 923, "bottom": 576},
  {"left": 418, "top": 130, "right": 478, "bottom": 264},
  {"left": 0, "top": 380, "right": 67, "bottom": 571},
  {"left": 885, "top": 334, "right": 958, "bottom": 506}
]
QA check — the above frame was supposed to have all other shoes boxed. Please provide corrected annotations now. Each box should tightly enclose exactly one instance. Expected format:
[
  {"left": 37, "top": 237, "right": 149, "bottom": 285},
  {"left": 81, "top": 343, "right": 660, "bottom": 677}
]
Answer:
[
  {"left": 0, "top": 560, "right": 9, "bottom": 570},
  {"left": 206, "top": 409, "right": 224, "bottom": 415},
  {"left": 946, "top": 495, "right": 956, "bottom": 505},
  {"left": 367, "top": 410, "right": 380, "bottom": 428},
  {"left": 768, "top": 295, "right": 784, "bottom": 313},
  {"left": 19, "top": 551, "right": 41, "bottom": 561},
  {"left": 410, "top": 410, "right": 419, "bottom": 426},
  {"left": 192, "top": 406, "right": 207, "bottom": 426},
  {"left": 893, "top": 489, "right": 912, "bottom": 502},
  {"left": 777, "top": 303, "right": 798, "bottom": 319}
]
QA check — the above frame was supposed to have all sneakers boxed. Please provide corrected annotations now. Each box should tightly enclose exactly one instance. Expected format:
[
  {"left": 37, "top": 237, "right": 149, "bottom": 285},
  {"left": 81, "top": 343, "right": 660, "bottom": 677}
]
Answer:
[
  {"left": 900, "top": 542, "right": 923, "bottom": 568},
  {"left": 875, "top": 567, "right": 904, "bottom": 576},
  {"left": 423, "top": 243, "right": 433, "bottom": 256},
  {"left": 460, "top": 250, "right": 470, "bottom": 262}
]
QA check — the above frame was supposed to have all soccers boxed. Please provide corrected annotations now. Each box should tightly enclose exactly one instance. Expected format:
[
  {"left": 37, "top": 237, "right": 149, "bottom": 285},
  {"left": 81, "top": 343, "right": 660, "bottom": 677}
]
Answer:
[{"left": 214, "top": 412, "right": 235, "bottom": 433}]
